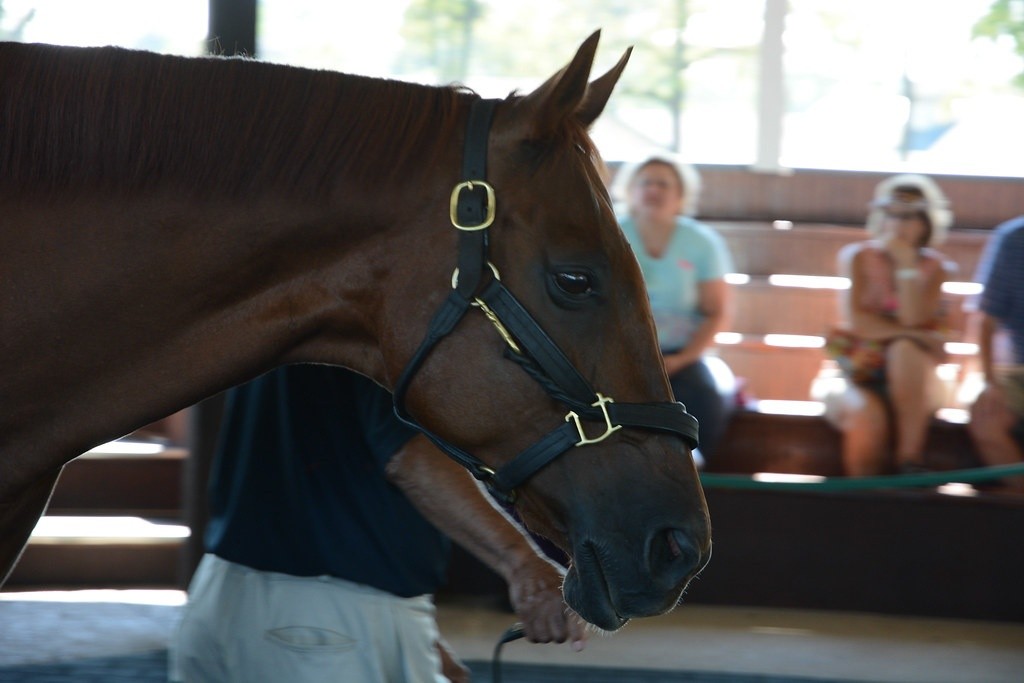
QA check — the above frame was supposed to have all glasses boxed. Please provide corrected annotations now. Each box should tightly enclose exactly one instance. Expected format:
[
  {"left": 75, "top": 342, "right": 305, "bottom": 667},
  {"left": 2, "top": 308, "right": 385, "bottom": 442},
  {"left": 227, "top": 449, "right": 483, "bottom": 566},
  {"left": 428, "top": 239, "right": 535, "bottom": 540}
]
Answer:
[{"left": 881, "top": 209, "right": 923, "bottom": 221}]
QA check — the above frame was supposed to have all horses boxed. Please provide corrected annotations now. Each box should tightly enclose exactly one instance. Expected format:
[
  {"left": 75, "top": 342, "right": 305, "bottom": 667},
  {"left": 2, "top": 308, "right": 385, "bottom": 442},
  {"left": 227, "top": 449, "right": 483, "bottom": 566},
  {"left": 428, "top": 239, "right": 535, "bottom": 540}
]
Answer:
[{"left": 0, "top": 27, "right": 714, "bottom": 634}]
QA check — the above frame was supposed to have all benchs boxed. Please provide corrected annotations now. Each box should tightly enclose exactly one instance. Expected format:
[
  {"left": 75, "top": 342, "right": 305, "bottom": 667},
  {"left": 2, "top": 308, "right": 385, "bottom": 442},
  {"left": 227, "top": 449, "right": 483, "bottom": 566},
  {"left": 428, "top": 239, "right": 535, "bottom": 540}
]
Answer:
[{"left": 692, "top": 217, "right": 996, "bottom": 479}]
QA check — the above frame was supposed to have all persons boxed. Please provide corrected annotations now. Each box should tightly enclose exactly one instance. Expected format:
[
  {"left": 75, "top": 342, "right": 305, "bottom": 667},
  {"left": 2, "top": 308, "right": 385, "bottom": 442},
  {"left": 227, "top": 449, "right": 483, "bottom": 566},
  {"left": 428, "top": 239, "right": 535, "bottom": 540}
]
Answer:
[
  {"left": 168, "top": 361, "right": 585, "bottom": 683},
  {"left": 617, "top": 156, "right": 735, "bottom": 469},
  {"left": 960, "top": 215, "right": 1024, "bottom": 487},
  {"left": 811, "top": 173, "right": 952, "bottom": 479}
]
